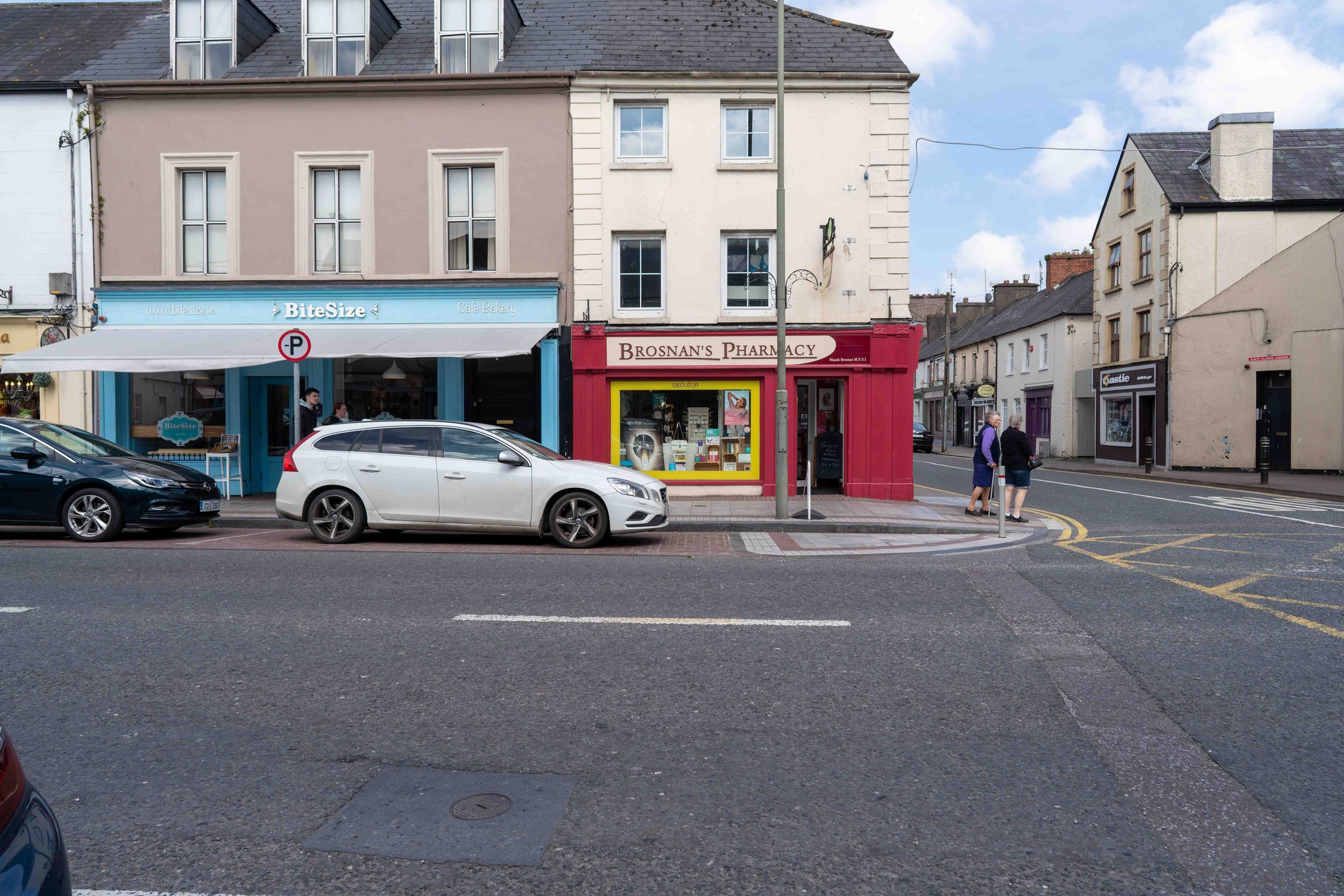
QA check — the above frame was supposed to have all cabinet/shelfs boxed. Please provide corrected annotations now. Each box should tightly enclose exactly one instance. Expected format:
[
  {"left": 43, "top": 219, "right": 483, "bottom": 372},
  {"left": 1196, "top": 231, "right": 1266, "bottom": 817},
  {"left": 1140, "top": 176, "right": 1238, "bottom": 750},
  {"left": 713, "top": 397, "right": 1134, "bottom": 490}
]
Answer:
[
  {"left": 720, "top": 437, "right": 745, "bottom": 471},
  {"left": 694, "top": 445, "right": 721, "bottom": 471}
]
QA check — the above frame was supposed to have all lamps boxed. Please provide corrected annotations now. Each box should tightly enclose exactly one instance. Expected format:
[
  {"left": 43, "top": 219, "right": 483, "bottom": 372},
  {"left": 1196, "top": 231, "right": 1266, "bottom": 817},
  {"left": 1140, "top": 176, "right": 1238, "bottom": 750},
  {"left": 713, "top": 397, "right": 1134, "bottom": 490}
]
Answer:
[
  {"left": 383, "top": 357, "right": 406, "bottom": 378},
  {"left": 183, "top": 370, "right": 209, "bottom": 379},
  {"left": 2, "top": 373, "right": 40, "bottom": 402}
]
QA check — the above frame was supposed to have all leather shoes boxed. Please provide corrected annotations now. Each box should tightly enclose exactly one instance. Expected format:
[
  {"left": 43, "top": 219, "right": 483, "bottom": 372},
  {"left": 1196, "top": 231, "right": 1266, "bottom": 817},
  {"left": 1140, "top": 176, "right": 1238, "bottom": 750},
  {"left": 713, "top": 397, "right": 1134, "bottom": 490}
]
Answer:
[
  {"left": 965, "top": 508, "right": 982, "bottom": 516},
  {"left": 980, "top": 508, "right": 997, "bottom": 516}
]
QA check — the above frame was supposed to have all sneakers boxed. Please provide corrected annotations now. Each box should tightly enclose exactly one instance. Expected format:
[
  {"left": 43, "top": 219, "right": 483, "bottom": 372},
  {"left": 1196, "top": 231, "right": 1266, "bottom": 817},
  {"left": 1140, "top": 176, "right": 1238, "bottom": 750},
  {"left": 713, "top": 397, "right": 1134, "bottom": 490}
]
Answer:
[
  {"left": 1011, "top": 514, "right": 1029, "bottom": 522},
  {"left": 1006, "top": 514, "right": 1012, "bottom": 521}
]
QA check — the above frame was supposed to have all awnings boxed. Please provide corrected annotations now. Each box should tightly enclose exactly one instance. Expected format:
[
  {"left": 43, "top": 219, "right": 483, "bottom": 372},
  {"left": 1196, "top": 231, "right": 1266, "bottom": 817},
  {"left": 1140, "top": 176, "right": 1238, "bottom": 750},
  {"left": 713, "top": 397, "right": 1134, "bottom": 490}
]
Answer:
[
  {"left": 193, "top": 387, "right": 224, "bottom": 400},
  {"left": 0, "top": 326, "right": 558, "bottom": 373}
]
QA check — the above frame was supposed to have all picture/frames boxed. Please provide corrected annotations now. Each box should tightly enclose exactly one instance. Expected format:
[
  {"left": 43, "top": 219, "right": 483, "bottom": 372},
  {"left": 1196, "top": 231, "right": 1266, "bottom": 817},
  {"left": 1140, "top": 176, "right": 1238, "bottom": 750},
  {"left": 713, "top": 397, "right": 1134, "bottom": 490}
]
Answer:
[{"left": 818, "top": 388, "right": 835, "bottom": 411}]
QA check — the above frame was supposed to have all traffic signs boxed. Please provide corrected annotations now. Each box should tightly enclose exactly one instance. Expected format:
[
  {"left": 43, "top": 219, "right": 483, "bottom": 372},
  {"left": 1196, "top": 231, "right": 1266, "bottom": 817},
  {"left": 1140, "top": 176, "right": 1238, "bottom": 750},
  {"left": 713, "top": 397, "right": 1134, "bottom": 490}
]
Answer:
[{"left": 278, "top": 329, "right": 311, "bottom": 362}]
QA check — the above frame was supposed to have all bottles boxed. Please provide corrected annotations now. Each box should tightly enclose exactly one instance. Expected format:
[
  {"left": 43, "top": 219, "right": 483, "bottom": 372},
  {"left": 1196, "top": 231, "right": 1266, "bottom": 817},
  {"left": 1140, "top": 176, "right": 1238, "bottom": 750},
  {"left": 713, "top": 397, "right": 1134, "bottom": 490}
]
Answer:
[
  {"left": 715, "top": 451, "right": 718, "bottom": 462},
  {"left": 709, "top": 451, "right": 712, "bottom": 462},
  {"left": 675, "top": 456, "right": 678, "bottom": 460},
  {"left": 712, "top": 451, "right": 715, "bottom": 462},
  {"left": 678, "top": 456, "right": 679, "bottom": 459},
  {"left": 641, "top": 435, "right": 649, "bottom": 468},
  {"left": 680, "top": 456, "right": 683, "bottom": 459}
]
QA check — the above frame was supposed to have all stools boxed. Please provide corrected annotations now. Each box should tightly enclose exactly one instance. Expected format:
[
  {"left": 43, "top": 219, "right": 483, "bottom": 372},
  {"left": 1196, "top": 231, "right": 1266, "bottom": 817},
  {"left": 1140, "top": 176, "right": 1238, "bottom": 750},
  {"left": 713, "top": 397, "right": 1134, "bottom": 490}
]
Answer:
[{"left": 206, "top": 434, "right": 243, "bottom": 500}]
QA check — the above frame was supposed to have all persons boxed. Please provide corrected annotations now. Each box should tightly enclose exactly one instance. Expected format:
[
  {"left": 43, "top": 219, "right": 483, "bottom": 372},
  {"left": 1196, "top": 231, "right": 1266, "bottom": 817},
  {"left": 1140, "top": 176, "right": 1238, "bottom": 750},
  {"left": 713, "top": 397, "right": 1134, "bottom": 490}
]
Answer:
[
  {"left": 24, "top": 415, "right": 33, "bottom": 420},
  {"left": 724, "top": 392, "right": 749, "bottom": 421},
  {"left": 1000, "top": 414, "right": 1035, "bottom": 523},
  {"left": 964, "top": 410, "right": 1002, "bottom": 516},
  {"left": 289, "top": 388, "right": 322, "bottom": 443},
  {"left": 322, "top": 401, "right": 347, "bottom": 425}
]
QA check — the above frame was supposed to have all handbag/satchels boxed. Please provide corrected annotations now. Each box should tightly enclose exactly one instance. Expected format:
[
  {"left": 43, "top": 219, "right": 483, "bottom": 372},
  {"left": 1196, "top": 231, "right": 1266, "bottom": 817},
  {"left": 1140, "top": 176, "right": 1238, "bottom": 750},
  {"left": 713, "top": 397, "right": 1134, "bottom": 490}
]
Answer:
[
  {"left": 670, "top": 422, "right": 687, "bottom": 451},
  {"left": 972, "top": 483, "right": 994, "bottom": 500},
  {"left": 1028, "top": 455, "right": 1043, "bottom": 470}
]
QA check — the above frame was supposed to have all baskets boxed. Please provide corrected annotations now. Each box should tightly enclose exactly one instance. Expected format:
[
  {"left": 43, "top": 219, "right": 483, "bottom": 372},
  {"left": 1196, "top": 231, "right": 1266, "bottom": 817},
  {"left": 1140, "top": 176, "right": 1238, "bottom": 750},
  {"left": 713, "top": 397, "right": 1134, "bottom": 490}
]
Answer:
[{"left": 210, "top": 443, "right": 236, "bottom": 453}]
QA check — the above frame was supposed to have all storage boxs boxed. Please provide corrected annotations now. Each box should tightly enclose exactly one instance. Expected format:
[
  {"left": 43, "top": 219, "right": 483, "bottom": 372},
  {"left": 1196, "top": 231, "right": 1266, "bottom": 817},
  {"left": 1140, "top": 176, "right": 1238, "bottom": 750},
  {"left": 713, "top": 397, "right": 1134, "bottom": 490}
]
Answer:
[{"left": 705, "top": 428, "right": 720, "bottom": 445}]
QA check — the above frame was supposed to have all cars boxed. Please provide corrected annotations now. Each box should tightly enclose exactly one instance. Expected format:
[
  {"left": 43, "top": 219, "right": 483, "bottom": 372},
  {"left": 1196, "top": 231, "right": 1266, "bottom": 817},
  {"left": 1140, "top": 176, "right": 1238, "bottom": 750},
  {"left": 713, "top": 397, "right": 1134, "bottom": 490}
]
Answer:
[
  {"left": 913, "top": 422, "right": 933, "bottom": 454},
  {"left": 1107, "top": 419, "right": 1129, "bottom": 442},
  {"left": 274, "top": 418, "right": 672, "bottom": 550},
  {"left": 0, "top": 416, "right": 221, "bottom": 542}
]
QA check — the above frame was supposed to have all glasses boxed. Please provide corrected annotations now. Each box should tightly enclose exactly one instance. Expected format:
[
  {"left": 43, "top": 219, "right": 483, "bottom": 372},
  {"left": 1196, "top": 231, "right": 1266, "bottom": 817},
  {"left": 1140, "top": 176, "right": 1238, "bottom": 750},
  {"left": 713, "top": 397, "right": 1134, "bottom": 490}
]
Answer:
[{"left": 992, "top": 419, "right": 1002, "bottom": 422}]
